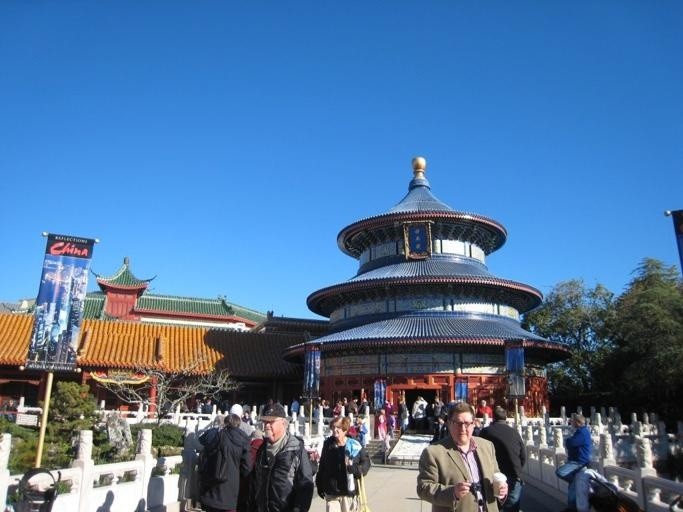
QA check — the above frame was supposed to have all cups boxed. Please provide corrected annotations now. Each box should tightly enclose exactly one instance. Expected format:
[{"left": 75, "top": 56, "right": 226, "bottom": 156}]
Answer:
[{"left": 492, "top": 472, "right": 508, "bottom": 498}]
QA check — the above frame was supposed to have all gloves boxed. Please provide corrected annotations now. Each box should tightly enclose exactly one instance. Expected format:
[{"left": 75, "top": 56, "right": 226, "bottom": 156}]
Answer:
[
  {"left": 317, "top": 488, "right": 325, "bottom": 499},
  {"left": 346, "top": 465, "right": 358, "bottom": 475}
]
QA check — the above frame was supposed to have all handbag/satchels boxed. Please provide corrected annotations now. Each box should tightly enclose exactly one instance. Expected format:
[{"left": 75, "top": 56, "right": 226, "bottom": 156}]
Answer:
[{"left": 200, "top": 431, "right": 228, "bottom": 489}]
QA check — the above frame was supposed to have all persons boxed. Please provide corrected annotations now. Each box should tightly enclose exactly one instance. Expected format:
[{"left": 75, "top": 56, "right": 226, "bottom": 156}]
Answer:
[
  {"left": 0, "top": 399, "right": 18, "bottom": 421},
  {"left": 555, "top": 415, "right": 593, "bottom": 512},
  {"left": 394, "top": 394, "right": 529, "bottom": 512},
  {"left": 160, "top": 395, "right": 398, "bottom": 512}
]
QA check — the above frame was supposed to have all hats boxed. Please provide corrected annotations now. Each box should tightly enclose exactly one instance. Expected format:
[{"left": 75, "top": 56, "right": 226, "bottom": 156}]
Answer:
[
  {"left": 229, "top": 403, "right": 242, "bottom": 417},
  {"left": 254, "top": 404, "right": 286, "bottom": 422}
]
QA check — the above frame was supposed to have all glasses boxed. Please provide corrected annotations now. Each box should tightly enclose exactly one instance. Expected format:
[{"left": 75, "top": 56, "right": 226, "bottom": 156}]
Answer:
[{"left": 449, "top": 419, "right": 473, "bottom": 429}]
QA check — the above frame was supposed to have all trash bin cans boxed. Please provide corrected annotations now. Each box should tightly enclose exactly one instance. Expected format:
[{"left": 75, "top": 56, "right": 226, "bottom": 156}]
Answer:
[{"left": 15, "top": 467, "right": 61, "bottom": 512}]
